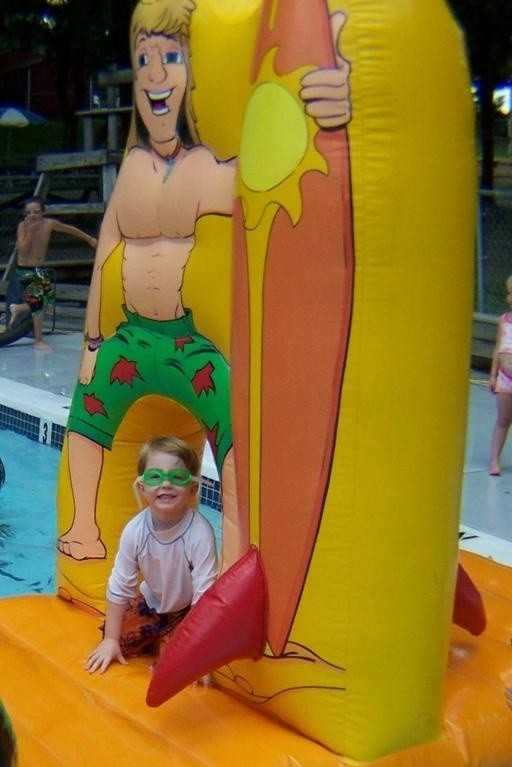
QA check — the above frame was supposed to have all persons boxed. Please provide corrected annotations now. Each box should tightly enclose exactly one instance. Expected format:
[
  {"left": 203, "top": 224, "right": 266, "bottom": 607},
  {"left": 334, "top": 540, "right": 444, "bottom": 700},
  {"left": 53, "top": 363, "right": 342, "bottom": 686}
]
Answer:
[
  {"left": 8, "top": 194, "right": 99, "bottom": 354},
  {"left": 82, "top": 435, "right": 220, "bottom": 690},
  {"left": 57, "top": 2, "right": 352, "bottom": 564},
  {"left": 486, "top": 276, "right": 511, "bottom": 477}
]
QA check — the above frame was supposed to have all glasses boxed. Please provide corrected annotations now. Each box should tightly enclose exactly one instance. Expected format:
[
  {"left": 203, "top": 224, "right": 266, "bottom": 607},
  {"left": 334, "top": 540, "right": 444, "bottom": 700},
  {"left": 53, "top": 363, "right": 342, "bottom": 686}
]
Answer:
[
  {"left": 132, "top": 467, "right": 216, "bottom": 511},
  {"left": 24, "top": 208, "right": 40, "bottom": 215}
]
